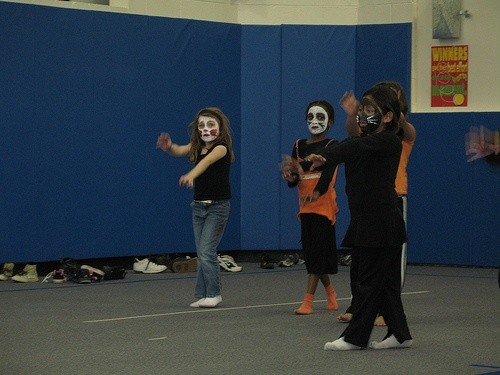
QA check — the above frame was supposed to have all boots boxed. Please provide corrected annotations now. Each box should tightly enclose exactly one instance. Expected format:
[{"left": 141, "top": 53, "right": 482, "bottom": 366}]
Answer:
[
  {"left": 0, "top": 263, "right": 14, "bottom": 280},
  {"left": 11, "top": 265, "right": 39, "bottom": 282}
]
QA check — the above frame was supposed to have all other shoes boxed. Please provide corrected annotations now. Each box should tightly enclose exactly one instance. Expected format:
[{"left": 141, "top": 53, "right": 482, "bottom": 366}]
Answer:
[
  {"left": 63, "top": 259, "right": 91, "bottom": 284},
  {"left": 339, "top": 251, "right": 352, "bottom": 266},
  {"left": 103, "top": 264, "right": 127, "bottom": 280},
  {"left": 168, "top": 256, "right": 197, "bottom": 273}
]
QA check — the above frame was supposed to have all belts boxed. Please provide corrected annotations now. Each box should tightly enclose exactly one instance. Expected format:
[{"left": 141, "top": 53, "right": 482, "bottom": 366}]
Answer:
[{"left": 193, "top": 199, "right": 212, "bottom": 204}]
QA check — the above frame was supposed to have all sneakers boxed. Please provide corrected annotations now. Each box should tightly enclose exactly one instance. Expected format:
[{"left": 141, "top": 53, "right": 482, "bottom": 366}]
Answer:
[
  {"left": 279, "top": 255, "right": 294, "bottom": 267},
  {"left": 132, "top": 257, "right": 167, "bottom": 274},
  {"left": 217, "top": 253, "right": 243, "bottom": 272},
  {"left": 294, "top": 254, "right": 304, "bottom": 265},
  {"left": 79, "top": 265, "right": 105, "bottom": 282},
  {"left": 40, "top": 268, "right": 66, "bottom": 283}
]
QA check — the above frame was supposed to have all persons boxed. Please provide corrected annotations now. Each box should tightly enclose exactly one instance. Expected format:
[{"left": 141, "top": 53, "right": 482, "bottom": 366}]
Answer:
[
  {"left": 279, "top": 100, "right": 338, "bottom": 313},
  {"left": 304, "top": 87, "right": 413, "bottom": 350},
  {"left": 463, "top": 126, "right": 500, "bottom": 164},
  {"left": 337, "top": 82, "right": 416, "bottom": 324},
  {"left": 156, "top": 107, "right": 233, "bottom": 308}
]
